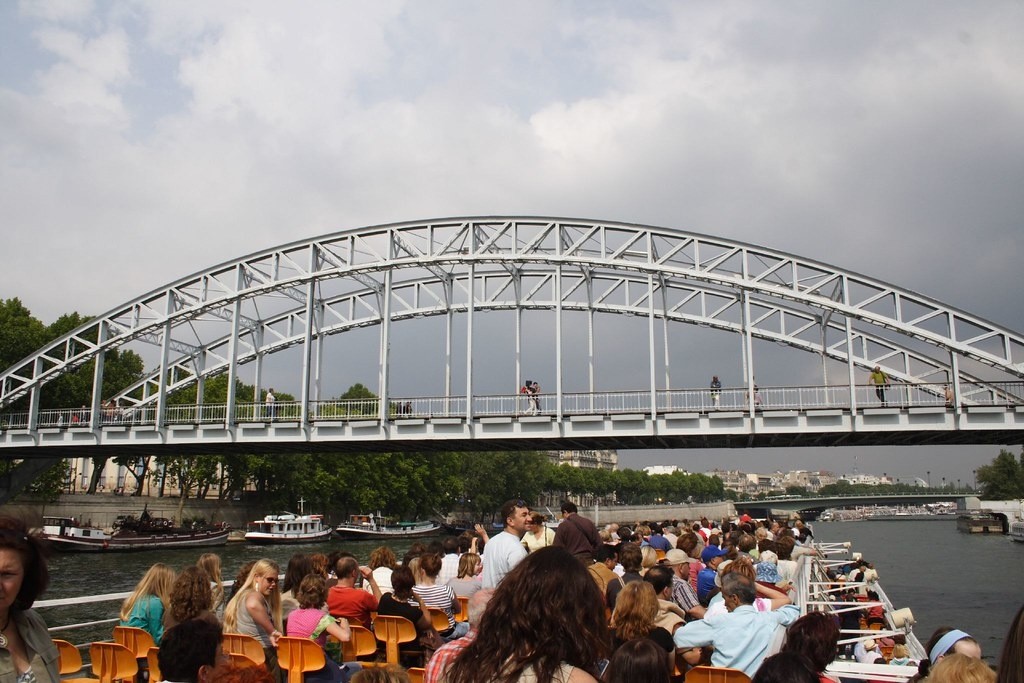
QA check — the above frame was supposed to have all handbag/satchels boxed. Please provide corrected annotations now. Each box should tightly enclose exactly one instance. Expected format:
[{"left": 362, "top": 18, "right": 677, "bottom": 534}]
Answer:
[{"left": 324, "top": 640, "right": 343, "bottom": 665}]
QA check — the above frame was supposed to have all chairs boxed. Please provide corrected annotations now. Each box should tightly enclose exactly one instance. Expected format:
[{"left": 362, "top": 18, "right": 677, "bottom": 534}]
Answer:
[
  {"left": 685, "top": 666, "right": 753, "bottom": 683},
  {"left": 52, "top": 597, "right": 468, "bottom": 683}
]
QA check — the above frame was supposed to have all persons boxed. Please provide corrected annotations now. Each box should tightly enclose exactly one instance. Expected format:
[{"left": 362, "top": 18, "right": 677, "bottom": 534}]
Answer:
[
  {"left": 395, "top": 401, "right": 412, "bottom": 416},
  {"left": 265, "top": 388, "right": 275, "bottom": 418},
  {"left": 57, "top": 398, "right": 125, "bottom": 427},
  {"left": 869, "top": 366, "right": 890, "bottom": 408},
  {"left": 710, "top": 376, "right": 722, "bottom": 411},
  {"left": 943, "top": 384, "right": 954, "bottom": 407},
  {"left": 747, "top": 376, "right": 764, "bottom": 408},
  {"left": 524, "top": 380, "right": 542, "bottom": 416},
  {"left": 0, "top": 498, "right": 1024, "bottom": 683}
]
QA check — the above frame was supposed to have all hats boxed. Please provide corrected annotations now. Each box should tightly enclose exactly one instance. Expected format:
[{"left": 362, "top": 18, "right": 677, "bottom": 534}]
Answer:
[
  {"left": 664, "top": 549, "right": 697, "bottom": 564},
  {"left": 714, "top": 559, "right": 734, "bottom": 588},
  {"left": 700, "top": 545, "right": 729, "bottom": 562},
  {"left": 754, "top": 562, "right": 783, "bottom": 583}
]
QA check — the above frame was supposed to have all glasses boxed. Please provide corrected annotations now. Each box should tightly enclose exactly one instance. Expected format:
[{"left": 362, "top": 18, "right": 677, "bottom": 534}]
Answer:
[
  {"left": 507, "top": 500, "right": 527, "bottom": 517},
  {"left": 261, "top": 575, "right": 279, "bottom": 583}
]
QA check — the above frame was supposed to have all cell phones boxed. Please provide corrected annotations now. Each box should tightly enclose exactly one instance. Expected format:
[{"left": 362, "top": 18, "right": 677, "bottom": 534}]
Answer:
[{"left": 595, "top": 658, "right": 609, "bottom": 680}]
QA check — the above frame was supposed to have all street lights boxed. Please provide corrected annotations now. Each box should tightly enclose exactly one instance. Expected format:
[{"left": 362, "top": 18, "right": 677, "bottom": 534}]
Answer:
[
  {"left": 973, "top": 469, "right": 976, "bottom": 493},
  {"left": 958, "top": 478, "right": 960, "bottom": 493},
  {"left": 943, "top": 477, "right": 945, "bottom": 490},
  {"left": 927, "top": 471, "right": 931, "bottom": 492}
]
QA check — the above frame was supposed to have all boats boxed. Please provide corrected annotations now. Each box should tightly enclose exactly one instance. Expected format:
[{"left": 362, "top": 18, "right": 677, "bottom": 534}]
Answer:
[
  {"left": 541, "top": 514, "right": 564, "bottom": 530},
  {"left": 1008, "top": 522, "right": 1024, "bottom": 542},
  {"left": 38, "top": 516, "right": 230, "bottom": 551},
  {"left": 822, "top": 508, "right": 948, "bottom": 521},
  {"left": 336, "top": 514, "right": 444, "bottom": 538},
  {"left": 442, "top": 520, "right": 504, "bottom": 534},
  {"left": 243, "top": 495, "right": 335, "bottom": 546}
]
